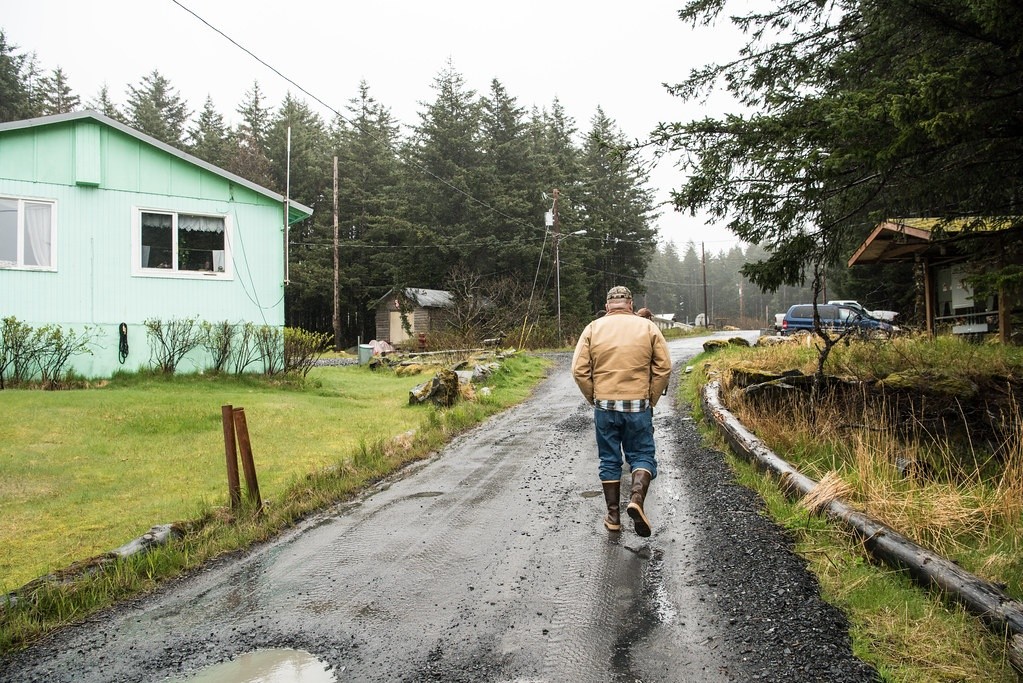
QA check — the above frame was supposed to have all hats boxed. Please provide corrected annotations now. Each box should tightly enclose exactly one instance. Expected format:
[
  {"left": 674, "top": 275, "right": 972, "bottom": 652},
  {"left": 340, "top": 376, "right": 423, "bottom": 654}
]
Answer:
[
  {"left": 637, "top": 308, "right": 654, "bottom": 317},
  {"left": 607, "top": 285, "right": 632, "bottom": 300}
]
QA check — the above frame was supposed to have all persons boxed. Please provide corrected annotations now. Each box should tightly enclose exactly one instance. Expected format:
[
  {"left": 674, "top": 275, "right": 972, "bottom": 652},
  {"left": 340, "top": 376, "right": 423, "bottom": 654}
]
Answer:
[
  {"left": 637, "top": 308, "right": 653, "bottom": 320},
  {"left": 572, "top": 286, "right": 671, "bottom": 537}
]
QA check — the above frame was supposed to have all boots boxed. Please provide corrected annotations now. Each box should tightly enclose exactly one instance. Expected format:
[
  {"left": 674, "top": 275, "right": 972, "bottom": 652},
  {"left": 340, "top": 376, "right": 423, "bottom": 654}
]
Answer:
[
  {"left": 626, "top": 468, "right": 654, "bottom": 538},
  {"left": 600, "top": 480, "right": 623, "bottom": 531}
]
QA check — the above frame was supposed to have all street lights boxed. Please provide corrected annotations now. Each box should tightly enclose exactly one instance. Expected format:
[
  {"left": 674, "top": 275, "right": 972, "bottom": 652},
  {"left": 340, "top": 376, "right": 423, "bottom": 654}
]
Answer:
[{"left": 556, "top": 230, "right": 588, "bottom": 343}]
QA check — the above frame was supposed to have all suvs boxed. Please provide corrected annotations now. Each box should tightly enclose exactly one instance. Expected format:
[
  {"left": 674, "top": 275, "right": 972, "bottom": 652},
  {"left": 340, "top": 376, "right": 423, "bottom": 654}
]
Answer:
[{"left": 782, "top": 304, "right": 893, "bottom": 339}]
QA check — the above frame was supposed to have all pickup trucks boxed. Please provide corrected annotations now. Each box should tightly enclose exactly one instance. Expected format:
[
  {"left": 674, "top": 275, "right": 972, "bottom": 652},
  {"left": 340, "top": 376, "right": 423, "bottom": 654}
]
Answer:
[{"left": 774, "top": 299, "right": 900, "bottom": 334}]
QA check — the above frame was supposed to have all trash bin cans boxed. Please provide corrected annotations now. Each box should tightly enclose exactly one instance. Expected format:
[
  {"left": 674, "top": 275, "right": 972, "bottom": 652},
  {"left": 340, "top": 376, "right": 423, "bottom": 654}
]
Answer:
[{"left": 359, "top": 344, "right": 375, "bottom": 367}]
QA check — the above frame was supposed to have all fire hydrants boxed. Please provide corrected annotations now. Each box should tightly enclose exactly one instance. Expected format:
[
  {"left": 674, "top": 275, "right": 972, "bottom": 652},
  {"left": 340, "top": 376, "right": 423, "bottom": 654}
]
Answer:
[{"left": 418, "top": 333, "right": 426, "bottom": 353}]
